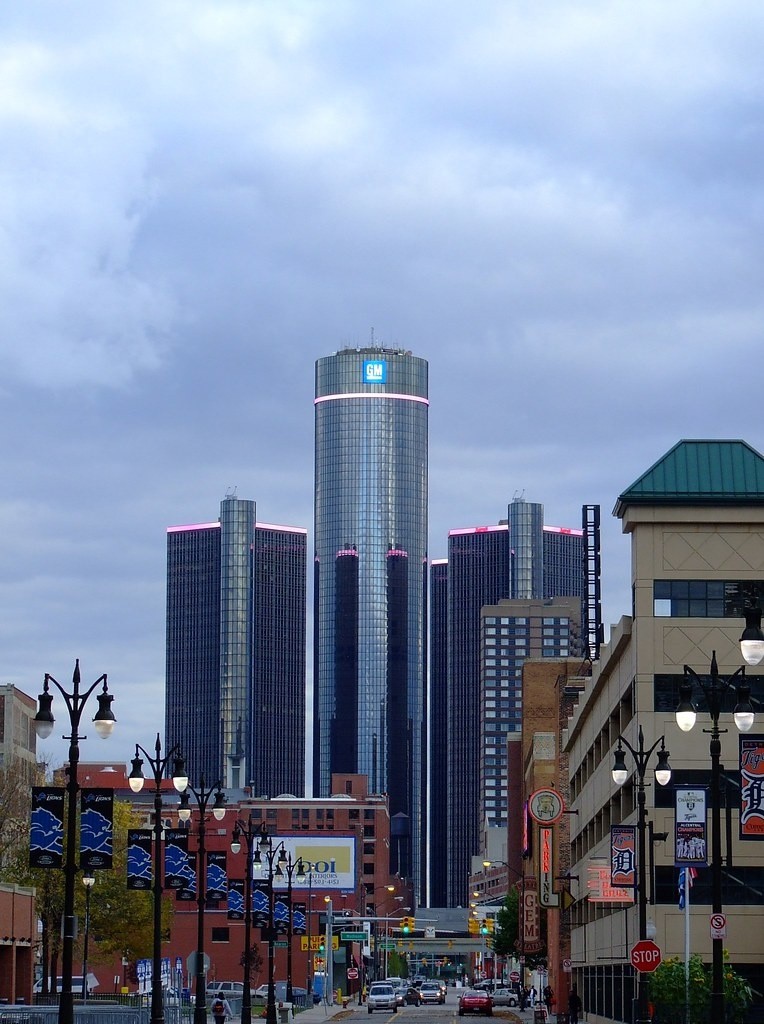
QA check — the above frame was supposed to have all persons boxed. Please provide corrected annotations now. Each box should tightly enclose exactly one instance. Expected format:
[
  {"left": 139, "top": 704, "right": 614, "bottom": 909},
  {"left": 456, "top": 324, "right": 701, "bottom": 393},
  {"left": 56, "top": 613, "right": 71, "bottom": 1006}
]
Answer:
[
  {"left": 543, "top": 986, "right": 554, "bottom": 1015},
  {"left": 524, "top": 986, "right": 528, "bottom": 1008},
  {"left": 569, "top": 989, "right": 583, "bottom": 1024},
  {"left": 676, "top": 837, "right": 705, "bottom": 859},
  {"left": 528, "top": 986, "right": 537, "bottom": 1008},
  {"left": 210, "top": 991, "right": 233, "bottom": 1024},
  {"left": 254, "top": 1004, "right": 267, "bottom": 1019}
]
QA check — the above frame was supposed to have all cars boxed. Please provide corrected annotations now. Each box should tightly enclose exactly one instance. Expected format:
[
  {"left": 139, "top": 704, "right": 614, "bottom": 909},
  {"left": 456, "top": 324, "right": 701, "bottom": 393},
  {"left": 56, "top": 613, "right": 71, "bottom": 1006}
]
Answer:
[
  {"left": 139, "top": 985, "right": 196, "bottom": 1007},
  {"left": 205, "top": 980, "right": 266, "bottom": 1002},
  {"left": 473, "top": 979, "right": 512, "bottom": 992},
  {"left": 366, "top": 974, "right": 448, "bottom": 1007},
  {"left": 268, "top": 983, "right": 322, "bottom": 1007},
  {"left": 365, "top": 984, "right": 398, "bottom": 1014},
  {"left": 457, "top": 989, "right": 494, "bottom": 1017},
  {"left": 490, "top": 988, "right": 520, "bottom": 1007}
]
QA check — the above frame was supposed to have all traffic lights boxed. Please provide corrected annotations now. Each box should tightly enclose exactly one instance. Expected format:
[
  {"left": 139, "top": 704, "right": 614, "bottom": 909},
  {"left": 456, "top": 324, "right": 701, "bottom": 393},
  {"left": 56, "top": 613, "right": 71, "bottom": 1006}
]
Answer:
[
  {"left": 403, "top": 916, "right": 409, "bottom": 933},
  {"left": 481, "top": 918, "right": 488, "bottom": 935},
  {"left": 468, "top": 917, "right": 475, "bottom": 934},
  {"left": 408, "top": 917, "right": 415, "bottom": 933},
  {"left": 487, "top": 918, "right": 495, "bottom": 934}
]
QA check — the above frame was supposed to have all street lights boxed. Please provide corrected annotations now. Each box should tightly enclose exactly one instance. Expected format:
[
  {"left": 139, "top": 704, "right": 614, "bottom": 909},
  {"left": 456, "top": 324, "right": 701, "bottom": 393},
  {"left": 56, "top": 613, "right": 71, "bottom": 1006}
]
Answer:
[
  {"left": 674, "top": 649, "right": 758, "bottom": 1024},
  {"left": 81, "top": 865, "right": 97, "bottom": 999},
  {"left": 357, "top": 884, "right": 395, "bottom": 1006},
  {"left": 252, "top": 835, "right": 289, "bottom": 1024},
  {"left": 127, "top": 731, "right": 190, "bottom": 1024},
  {"left": 175, "top": 777, "right": 228, "bottom": 1024},
  {"left": 32, "top": 657, "right": 117, "bottom": 1024},
  {"left": 374, "top": 896, "right": 403, "bottom": 983},
  {"left": 228, "top": 814, "right": 272, "bottom": 1024},
  {"left": 384, "top": 906, "right": 411, "bottom": 980},
  {"left": 609, "top": 723, "right": 672, "bottom": 1024},
  {"left": 468, "top": 890, "right": 504, "bottom": 988},
  {"left": 273, "top": 851, "right": 306, "bottom": 1020},
  {"left": 482, "top": 859, "right": 526, "bottom": 1013}
]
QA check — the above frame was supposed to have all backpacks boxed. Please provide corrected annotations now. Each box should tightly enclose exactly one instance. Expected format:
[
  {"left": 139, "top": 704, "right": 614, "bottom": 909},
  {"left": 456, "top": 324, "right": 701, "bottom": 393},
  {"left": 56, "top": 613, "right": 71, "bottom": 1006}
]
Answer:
[{"left": 213, "top": 1000, "right": 226, "bottom": 1015}]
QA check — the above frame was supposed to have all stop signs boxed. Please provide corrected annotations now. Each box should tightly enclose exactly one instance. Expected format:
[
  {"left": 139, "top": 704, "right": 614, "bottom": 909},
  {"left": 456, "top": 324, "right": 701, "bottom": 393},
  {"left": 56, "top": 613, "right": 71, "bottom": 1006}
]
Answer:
[
  {"left": 509, "top": 971, "right": 520, "bottom": 982},
  {"left": 630, "top": 940, "right": 662, "bottom": 973},
  {"left": 347, "top": 967, "right": 358, "bottom": 979}
]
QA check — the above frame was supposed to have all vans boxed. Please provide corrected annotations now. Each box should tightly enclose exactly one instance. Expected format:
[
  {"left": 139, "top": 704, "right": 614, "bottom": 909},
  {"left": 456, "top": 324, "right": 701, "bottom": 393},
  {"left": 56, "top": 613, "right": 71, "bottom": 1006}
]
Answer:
[{"left": 32, "top": 975, "right": 90, "bottom": 1000}]
